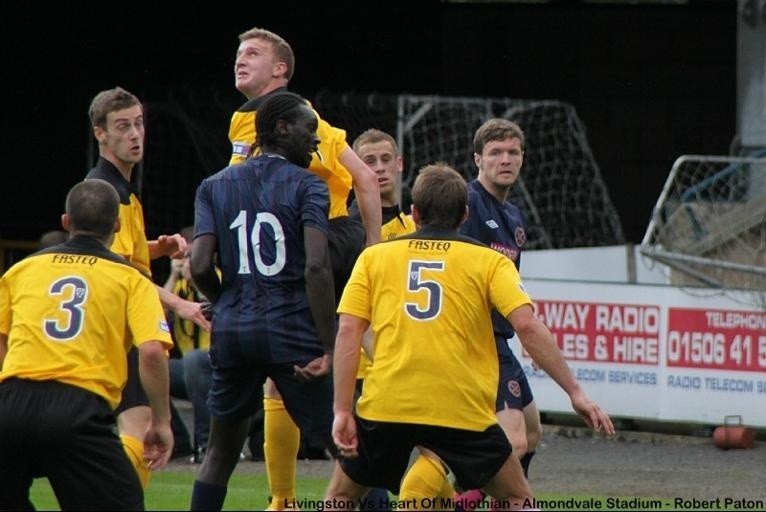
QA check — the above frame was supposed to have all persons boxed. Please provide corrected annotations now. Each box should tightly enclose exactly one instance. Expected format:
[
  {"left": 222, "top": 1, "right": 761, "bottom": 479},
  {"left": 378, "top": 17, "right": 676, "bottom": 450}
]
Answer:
[
  {"left": 153, "top": 225, "right": 243, "bottom": 465},
  {"left": 43, "top": 85, "right": 213, "bottom": 495},
  {"left": 342, "top": 121, "right": 457, "bottom": 511},
  {"left": 1, "top": 175, "right": 177, "bottom": 512},
  {"left": 322, "top": 162, "right": 618, "bottom": 511},
  {"left": 203, "top": 25, "right": 384, "bottom": 511},
  {"left": 188, "top": 86, "right": 345, "bottom": 511},
  {"left": 453, "top": 116, "right": 545, "bottom": 511}
]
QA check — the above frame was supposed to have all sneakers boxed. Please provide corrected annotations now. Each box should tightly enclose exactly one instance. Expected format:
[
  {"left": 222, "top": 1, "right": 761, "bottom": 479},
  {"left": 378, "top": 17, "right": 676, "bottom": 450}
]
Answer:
[{"left": 171, "top": 442, "right": 208, "bottom": 464}]
